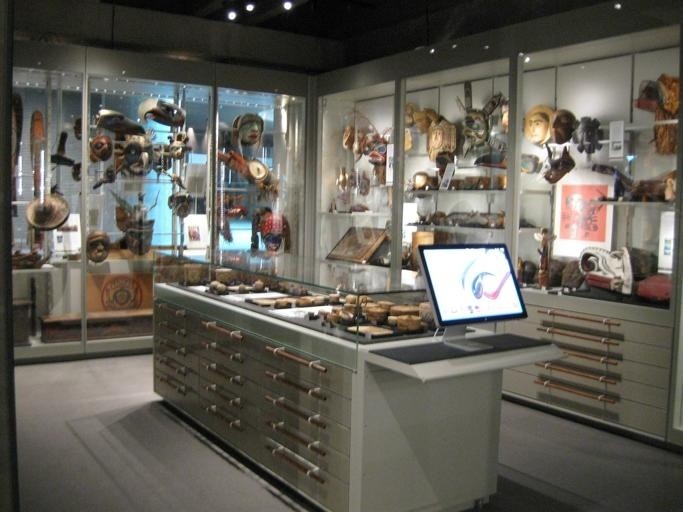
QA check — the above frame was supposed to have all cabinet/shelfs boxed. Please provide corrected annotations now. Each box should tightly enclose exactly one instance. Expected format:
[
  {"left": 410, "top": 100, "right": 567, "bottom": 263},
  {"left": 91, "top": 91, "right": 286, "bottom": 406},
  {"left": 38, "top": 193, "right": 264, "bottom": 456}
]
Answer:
[
  {"left": 315, "top": 21, "right": 680, "bottom": 448},
  {"left": 11, "top": 67, "right": 311, "bottom": 366},
  {"left": 152, "top": 249, "right": 568, "bottom": 512}
]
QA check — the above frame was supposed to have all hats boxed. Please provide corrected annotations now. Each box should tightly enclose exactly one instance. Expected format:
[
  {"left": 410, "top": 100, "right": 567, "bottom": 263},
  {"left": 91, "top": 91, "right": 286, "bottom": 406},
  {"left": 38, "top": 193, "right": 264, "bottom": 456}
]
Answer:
[{"left": 24, "top": 191, "right": 70, "bottom": 232}]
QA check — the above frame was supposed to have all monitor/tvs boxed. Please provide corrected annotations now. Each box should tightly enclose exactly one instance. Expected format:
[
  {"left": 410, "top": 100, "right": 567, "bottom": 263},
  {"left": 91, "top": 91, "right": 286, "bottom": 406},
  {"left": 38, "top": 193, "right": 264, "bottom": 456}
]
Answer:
[{"left": 415, "top": 243, "right": 529, "bottom": 353}]
